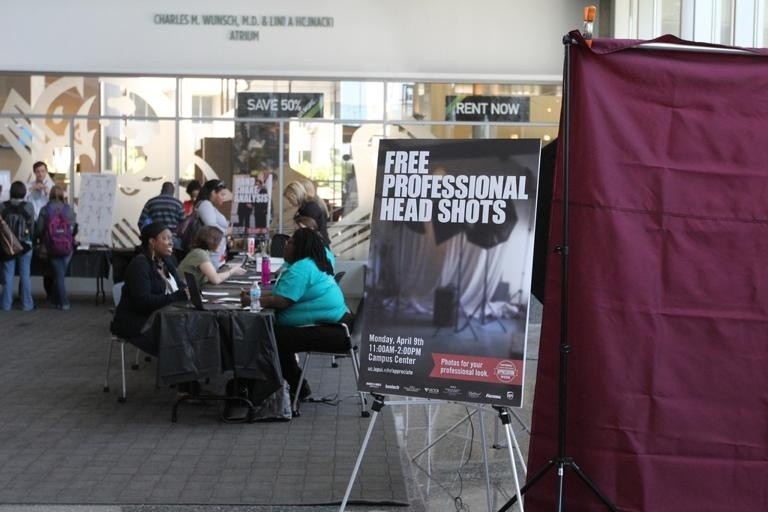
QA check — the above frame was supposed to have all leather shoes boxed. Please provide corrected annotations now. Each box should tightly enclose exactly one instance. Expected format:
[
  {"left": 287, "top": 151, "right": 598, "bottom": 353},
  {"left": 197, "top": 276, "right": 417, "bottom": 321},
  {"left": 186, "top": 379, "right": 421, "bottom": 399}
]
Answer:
[{"left": 295, "top": 384, "right": 310, "bottom": 399}]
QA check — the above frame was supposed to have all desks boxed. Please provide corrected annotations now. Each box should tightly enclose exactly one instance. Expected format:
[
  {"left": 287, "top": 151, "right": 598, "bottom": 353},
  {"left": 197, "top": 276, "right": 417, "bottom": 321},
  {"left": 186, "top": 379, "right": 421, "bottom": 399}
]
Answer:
[
  {"left": 15, "top": 245, "right": 114, "bottom": 307},
  {"left": 140, "top": 245, "right": 297, "bottom": 426},
  {"left": 106, "top": 246, "right": 137, "bottom": 280}
]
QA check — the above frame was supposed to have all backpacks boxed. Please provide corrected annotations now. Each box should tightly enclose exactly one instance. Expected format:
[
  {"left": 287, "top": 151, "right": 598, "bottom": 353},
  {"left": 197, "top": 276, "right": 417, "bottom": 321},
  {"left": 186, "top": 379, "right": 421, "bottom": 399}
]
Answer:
[
  {"left": 3, "top": 201, "right": 33, "bottom": 250},
  {"left": 44, "top": 204, "right": 74, "bottom": 256}
]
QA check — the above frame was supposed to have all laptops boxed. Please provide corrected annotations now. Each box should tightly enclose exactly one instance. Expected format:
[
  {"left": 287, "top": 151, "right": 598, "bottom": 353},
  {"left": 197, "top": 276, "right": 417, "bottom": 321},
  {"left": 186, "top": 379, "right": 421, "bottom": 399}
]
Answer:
[{"left": 184, "top": 271, "right": 242, "bottom": 311}]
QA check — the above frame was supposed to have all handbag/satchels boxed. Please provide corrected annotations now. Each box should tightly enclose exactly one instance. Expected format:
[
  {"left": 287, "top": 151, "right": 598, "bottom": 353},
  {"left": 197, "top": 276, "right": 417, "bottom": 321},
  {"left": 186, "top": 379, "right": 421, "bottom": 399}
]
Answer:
[
  {"left": 181, "top": 211, "right": 203, "bottom": 248},
  {"left": 223, "top": 378, "right": 292, "bottom": 421}
]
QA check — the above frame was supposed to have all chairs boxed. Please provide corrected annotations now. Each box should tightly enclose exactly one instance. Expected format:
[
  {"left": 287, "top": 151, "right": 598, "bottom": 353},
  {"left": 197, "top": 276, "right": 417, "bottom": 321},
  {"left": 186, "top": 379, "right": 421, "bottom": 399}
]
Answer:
[
  {"left": 292, "top": 263, "right": 373, "bottom": 420},
  {"left": 333, "top": 270, "right": 346, "bottom": 285},
  {"left": 103, "top": 282, "right": 145, "bottom": 408}
]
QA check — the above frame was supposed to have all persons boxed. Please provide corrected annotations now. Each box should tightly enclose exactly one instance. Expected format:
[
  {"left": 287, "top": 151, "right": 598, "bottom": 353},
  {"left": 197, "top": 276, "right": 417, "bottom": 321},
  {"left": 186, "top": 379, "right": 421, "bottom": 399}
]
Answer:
[
  {"left": 26, "top": 162, "right": 55, "bottom": 245},
  {"left": 240, "top": 179, "right": 354, "bottom": 406},
  {"left": 238, "top": 180, "right": 274, "bottom": 247},
  {"left": 34, "top": 186, "right": 78, "bottom": 310},
  {"left": 109, "top": 179, "right": 235, "bottom": 401},
  {"left": 0, "top": 182, "right": 36, "bottom": 312}
]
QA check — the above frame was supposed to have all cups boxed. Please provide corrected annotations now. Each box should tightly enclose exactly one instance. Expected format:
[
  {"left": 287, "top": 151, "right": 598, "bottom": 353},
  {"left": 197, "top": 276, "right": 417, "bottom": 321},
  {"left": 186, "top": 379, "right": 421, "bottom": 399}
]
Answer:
[{"left": 246, "top": 237, "right": 254, "bottom": 254}]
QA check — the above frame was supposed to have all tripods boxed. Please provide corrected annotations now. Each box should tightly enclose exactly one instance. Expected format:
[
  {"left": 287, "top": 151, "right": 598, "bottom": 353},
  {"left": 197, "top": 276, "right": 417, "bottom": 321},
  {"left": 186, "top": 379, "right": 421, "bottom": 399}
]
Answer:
[{"left": 498, "top": 40, "right": 620, "bottom": 512}]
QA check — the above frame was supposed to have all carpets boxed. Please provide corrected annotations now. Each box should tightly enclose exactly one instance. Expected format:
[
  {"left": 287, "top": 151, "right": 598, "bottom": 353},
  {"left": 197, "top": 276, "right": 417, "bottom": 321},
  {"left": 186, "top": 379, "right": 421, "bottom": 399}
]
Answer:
[{"left": 1, "top": 270, "right": 412, "bottom": 508}]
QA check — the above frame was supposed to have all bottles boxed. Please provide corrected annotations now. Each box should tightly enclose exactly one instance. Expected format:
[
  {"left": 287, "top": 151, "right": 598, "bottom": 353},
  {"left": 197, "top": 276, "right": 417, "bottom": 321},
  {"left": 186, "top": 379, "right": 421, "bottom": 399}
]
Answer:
[
  {"left": 261, "top": 254, "right": 271, "bottom": 285},
  {"left": 250, "top": 280, "right": 261, "bottom": 313}
]
